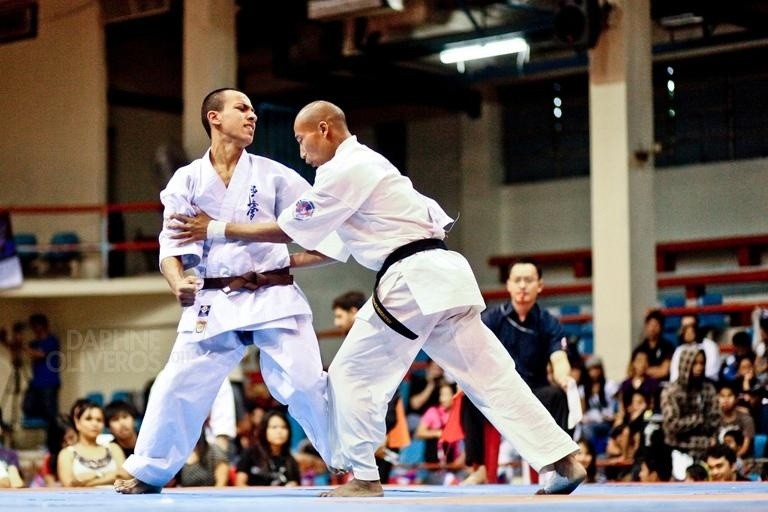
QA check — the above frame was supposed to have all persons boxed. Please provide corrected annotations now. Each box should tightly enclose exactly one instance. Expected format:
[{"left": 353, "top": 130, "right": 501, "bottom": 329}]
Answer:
[
  {"left": 111, "top": 86, "right": 354, "bottom": 495},
  {"left": 164, "top": 96, "right": 587, "bottom": 496},
  {"left": 0, "top": 259, "right": 766, "bottom": 488}
]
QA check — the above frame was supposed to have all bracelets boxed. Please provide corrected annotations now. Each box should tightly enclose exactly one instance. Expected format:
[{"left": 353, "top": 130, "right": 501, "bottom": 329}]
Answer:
[{"left": 204, "top": 218, "right": 231, "bottom": 244}]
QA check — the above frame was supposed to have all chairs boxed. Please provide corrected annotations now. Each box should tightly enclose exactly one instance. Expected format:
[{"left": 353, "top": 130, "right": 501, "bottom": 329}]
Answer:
[{"left": 15, "top": 231, "right": 82, "bottom": 276}]
